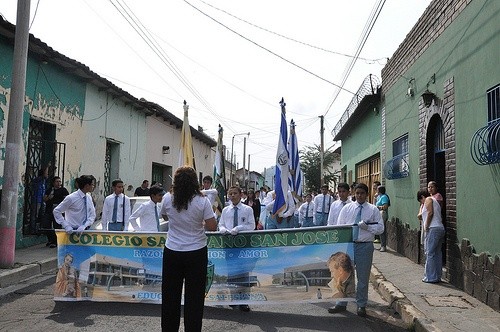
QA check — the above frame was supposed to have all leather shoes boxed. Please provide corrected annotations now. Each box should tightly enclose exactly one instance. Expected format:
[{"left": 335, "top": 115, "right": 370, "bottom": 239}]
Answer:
[
  {"left": 356, "top": 306, "right": 367, "bottom": 316},
  {"left": 228, "top": 305, "right": 250, "bottom": 312},
  {"left": 327, "top": 305, "right": 347, "bottom": 313}
]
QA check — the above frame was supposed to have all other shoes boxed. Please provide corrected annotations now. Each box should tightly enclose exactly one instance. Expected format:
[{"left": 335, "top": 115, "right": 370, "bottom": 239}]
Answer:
[
  {"left": 373, "top": 239, "right": 387, "bottom": 252},
  {"left": 46, "top": 241, "right": 57, "bottom": 247}
]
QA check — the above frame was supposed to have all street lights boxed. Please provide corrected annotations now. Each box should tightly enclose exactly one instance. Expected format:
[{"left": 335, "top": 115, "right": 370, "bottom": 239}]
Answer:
[{"left": 230, "top": 132, "right": 249, "bottom": 188}]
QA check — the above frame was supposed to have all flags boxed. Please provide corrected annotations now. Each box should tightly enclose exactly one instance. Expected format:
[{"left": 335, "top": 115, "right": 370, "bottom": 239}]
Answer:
[
  {"left": 216, "top": 133, "right": 228, "bottom": 204},
  {"left": 273, "top": 107, "right": 305, "bottom": 218},
  {"left": 180, "top": 113, "right": 195, "bottom": 170}
]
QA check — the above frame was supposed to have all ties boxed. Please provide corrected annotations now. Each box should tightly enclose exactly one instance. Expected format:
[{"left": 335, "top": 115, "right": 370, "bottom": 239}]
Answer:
[
  {"left": 233, "top": 207, "right": 239, "bottom": 228},
  {"left": 352, "top": 206, "right": 363, "bottom": 240},
  {"left": 305, "top": 204, "right": 310, "bottom": 220},
  {"left": 112, "top": 196, "right": 119, "bottom": 223},
  {"left": 155, "top": 206, "right": 161, "bottom": 232},
  {"left": 322, "top": 195, "right": 326, "bottom": 216},
  {"left": 83, "top": 196, "right": 87, "bottom": 223}
]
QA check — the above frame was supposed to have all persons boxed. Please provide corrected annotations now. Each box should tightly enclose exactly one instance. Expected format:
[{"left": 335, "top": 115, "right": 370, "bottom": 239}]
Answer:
[
  {"left": 328, "top": 182, "right": 385, "bottom": 316},
  {"left": 417, "top": 180, "right": 443, "bottom": 221},
  {"left": 34, "top": 173, "right": 390, "bottom": 255},
  {"left": 416, "top": 189, "right": 446, "bottom": 284},
  {"left": 159, "top": 166, "right": 218, "bottom": 332}
]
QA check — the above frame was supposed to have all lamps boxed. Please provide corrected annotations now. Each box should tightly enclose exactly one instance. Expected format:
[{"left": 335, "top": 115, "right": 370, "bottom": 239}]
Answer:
[{"left": 419, "top": 85, "right": 435, "bottom": 107}]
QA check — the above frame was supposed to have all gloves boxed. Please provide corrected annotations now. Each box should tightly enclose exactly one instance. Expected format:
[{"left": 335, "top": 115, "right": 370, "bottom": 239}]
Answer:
[
  {"left": 218, "top": 226, "right": 239, "bottom": 235},
  {"left": 65, "top": 225, "right": 73, "bottom": 234},
  {"left": 102, "top": 225, "right": 107, "bottom": 231},
  {"left": 76, "top": 226, "right": 86, "bottom": 237}
]
